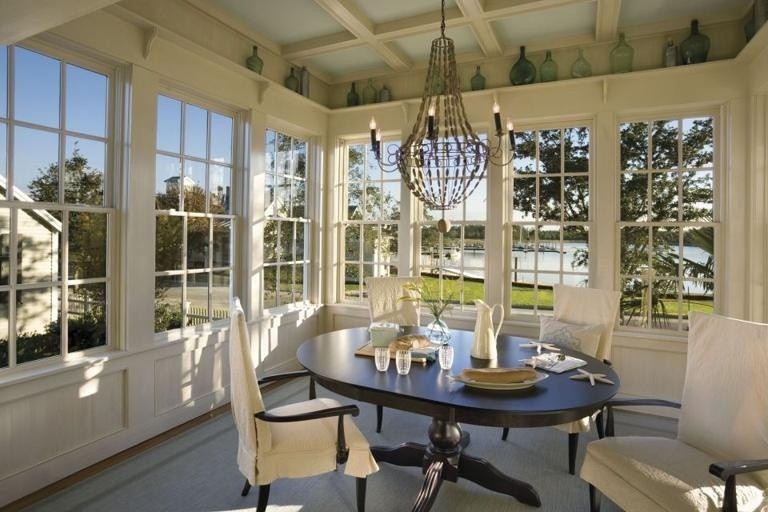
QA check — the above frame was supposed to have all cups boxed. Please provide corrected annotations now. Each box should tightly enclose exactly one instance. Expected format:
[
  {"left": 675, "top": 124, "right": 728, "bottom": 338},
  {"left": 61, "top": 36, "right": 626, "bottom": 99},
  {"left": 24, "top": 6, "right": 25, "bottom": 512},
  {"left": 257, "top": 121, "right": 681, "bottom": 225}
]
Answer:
[
  {"left": 438, "top": 345, "right": 454, "bottom": 371},
  {"left": 373, "top": 347, "right": 390, "bottom": 372},
  {"left": 394, "top": 349, "right": 412, "bottom": 376}
]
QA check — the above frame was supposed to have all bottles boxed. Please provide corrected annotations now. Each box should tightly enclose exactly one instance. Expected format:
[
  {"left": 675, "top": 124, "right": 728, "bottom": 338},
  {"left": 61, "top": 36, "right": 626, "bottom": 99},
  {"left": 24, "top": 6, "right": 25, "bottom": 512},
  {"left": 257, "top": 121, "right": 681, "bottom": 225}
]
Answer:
[
  {"left": 285, "top": 66, "right": 298, "bottom": 92},
  {"left": 569, "top": 46, "right": 592, "bottom": 79},
  {"left": 677, "top": 18, "right": 711, "bottom": 69},
  {"left": 300, "top": 66, "right": 311, "bottom": 97},
  {"left": 508, "top": 44, "right": 536, "bottom": 86},
  {"left": 245, "top": 45, "right": 263, "bottom": 74},
  {"left": 538, "top": 48, "right": 558, "bottom": 84},
  {"left": 469, "top": 65, "right": 485, "bottom": 92},
  {"left": 661, "top": 35, "right": 679, "bottom": 69},
  {"left": 608, "top": 30, "right": 634, "bottom": 74},
  {"left": 347, "top": 77, "right": 390, "bottom": 108}
]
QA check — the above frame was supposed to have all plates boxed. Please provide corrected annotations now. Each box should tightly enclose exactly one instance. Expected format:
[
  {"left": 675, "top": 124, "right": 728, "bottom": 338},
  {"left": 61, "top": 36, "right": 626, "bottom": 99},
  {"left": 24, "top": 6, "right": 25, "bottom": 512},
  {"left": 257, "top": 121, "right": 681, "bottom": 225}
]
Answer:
[{"left": 448, "top": 370, "right": 550, "bottom": 392}]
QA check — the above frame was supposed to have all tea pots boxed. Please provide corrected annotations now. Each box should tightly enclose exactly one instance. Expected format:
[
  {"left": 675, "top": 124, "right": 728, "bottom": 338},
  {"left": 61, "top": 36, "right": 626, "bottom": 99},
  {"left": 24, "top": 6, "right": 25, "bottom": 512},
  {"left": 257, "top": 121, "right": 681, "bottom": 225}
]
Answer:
[{"left": 469, "top": 297, "right": 503, "bottom": 360}]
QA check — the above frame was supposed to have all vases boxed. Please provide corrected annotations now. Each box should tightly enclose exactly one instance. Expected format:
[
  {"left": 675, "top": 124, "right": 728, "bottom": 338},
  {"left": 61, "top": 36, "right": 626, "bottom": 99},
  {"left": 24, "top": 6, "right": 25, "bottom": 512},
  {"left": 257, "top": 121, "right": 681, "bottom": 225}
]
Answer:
[
  {"left": 245, "top": 44, "right": 390, "bottom": 108},
  {"left": 509, "top": 17, "right": 711, "bottom": 85},
  {"left": 470, "top": 66, "right": 485, "bottom": 91}
]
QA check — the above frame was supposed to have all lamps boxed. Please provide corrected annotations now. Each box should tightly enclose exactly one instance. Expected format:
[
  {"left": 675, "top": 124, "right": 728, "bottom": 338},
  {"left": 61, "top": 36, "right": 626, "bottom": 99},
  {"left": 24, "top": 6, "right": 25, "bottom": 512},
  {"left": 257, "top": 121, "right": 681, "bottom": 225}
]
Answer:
[{"left": 368, "top": 0, "right": 516, "bottom": 234}]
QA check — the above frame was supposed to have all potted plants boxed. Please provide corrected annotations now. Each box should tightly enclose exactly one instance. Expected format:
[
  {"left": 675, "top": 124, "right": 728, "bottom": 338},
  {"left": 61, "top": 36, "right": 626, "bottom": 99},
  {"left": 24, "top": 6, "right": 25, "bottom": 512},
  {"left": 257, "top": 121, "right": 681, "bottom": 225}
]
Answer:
[{"left": 398, "top": 278, "right": 457, "bottom": 343}]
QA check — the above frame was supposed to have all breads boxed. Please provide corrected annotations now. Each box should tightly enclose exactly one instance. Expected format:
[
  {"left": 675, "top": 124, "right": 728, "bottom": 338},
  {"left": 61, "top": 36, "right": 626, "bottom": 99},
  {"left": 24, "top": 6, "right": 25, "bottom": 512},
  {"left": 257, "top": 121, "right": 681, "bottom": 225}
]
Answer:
[
  {"left": 463, "top": 368, "right": 534, "bottom": 384},
  {"left": 389, "top": 336, "right": 431, "bottom": 352}
]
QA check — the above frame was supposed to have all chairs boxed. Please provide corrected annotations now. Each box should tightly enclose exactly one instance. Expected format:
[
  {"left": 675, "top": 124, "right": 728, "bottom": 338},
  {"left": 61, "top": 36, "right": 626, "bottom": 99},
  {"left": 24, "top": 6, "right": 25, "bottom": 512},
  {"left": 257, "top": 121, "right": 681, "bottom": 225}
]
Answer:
[
  {"left": 366, "top": 277, "right": 422, "bottom": 431},
  {"left": 580, "top": 312, "right": 768, "bottom": 512},
  {"left": 226, "top": 295, "right": 379, "bottom": 510},
  {"left": 502, "top": 284, "right": 622, "bottom": 476}
]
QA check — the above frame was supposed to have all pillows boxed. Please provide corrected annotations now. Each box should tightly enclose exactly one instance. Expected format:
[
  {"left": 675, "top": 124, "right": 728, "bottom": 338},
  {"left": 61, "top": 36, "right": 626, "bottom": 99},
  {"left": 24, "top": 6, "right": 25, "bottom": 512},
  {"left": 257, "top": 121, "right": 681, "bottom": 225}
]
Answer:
[{"left": 537, "top": 315, "right": 602, "bottom": 357}]
